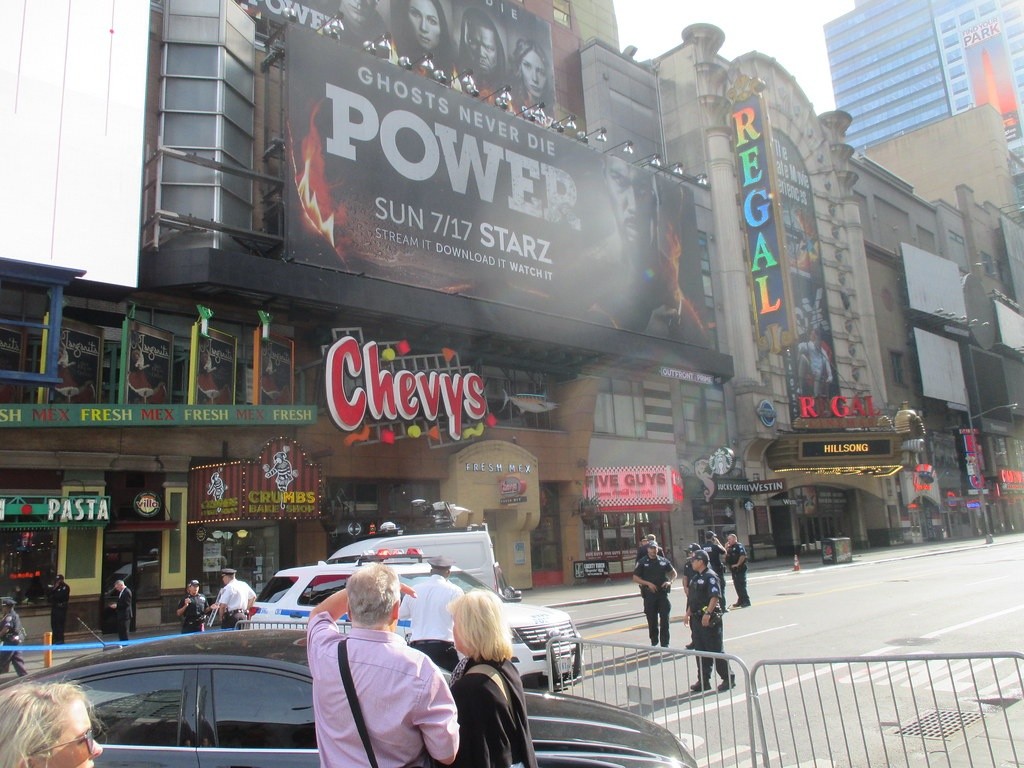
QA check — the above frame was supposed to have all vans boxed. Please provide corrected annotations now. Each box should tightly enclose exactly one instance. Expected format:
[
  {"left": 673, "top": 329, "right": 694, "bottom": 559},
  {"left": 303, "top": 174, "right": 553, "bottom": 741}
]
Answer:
[
  {"left": 324, "top": 500, "right": 523, "bottom": 603},
  {"left": 106, "top": 549, "right": 159, "bottom": 598}
]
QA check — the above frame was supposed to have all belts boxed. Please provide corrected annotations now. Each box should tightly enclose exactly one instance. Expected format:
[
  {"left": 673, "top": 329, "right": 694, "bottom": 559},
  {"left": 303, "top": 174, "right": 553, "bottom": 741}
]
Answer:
[{"left": 413, "top": 640, "right": 443, "bottom": 644}]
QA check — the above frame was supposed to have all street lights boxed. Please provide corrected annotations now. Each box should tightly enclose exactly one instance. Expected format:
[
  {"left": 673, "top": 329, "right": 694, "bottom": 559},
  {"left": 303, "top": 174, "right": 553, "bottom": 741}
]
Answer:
[{"left": 969, "top": 404, "right": 1018, "bottom": 544}]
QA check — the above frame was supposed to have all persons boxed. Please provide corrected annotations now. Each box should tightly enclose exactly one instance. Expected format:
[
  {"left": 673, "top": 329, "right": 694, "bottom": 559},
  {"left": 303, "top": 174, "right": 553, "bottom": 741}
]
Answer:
[
  {"left": 635, "top": 534, "right": 664, "bottom": 566},
  {"left": 399, "top": 557, "right": 465, "bottom": 673},
  {"left": 798, "top": 329, "right": 832, "bottom": 395},
  {"left": 702, "top": 531, "right": 728, "bottom": 612},
  {"left": 427, "top": 589, "right": 538, "bottom": 768},
  {"left": 340, "top": 0, "right": 554, "bottom": 119},
  {"left": 177, "top": 580, "right": 217, "bottom": 634},
  {"left": 111, "top": 580, "right": 133, "bottom": 641},
  {"left": 595, "top": 157, "right": 709, "bottom": 346},
  {"left": 217, "top": 569, "right": 257, "bottom": 629},
  {"left": 683, "top": 543, "right": 701, "bottom": 650},
  {"left": 684, "top": 550, "right": 736, "bottom": 691},
  {"left": 633, "top": 541, "right": 677, "bottom": 647},
  {"left": 306, "top": 562, "right": 461, "bottom": 768},
  {"left": 48, "top": 574, "right": 70, "bottom": 644},
  {"left": 725, "top": 534, "right": 751, "bottom": 607},
  {"left": 0, "top": 683, "right": 103, "bottom": 768},
  {"left": 0, "top": 598, "right": 28, "bottom": 676}
]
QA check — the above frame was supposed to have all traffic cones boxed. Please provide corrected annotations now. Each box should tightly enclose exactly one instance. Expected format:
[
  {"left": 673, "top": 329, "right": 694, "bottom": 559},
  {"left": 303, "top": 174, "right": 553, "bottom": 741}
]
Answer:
[{"left": 792, "top": 555, "right": 802, "bottom": 571}]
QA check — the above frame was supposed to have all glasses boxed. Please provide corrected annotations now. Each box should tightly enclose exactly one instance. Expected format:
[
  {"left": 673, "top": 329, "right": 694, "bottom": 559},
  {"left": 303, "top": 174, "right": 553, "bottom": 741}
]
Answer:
[{"left": 26, "top": 729, "right": 94, "bottom": 757}]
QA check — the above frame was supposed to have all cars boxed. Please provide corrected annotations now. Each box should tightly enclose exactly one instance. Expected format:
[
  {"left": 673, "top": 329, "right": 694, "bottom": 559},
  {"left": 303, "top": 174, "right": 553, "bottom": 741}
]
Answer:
[{"left": 5, "top": 629, "right": 700, "bottom": 768}]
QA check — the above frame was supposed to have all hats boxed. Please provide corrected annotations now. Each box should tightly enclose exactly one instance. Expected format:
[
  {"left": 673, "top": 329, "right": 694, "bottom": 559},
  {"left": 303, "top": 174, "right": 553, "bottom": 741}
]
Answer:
[
  {"left": 2, "top": 598, "right": 16, "bottom": 607},
  {"left": 219, "top": 568, "right": 237, "bottom": 577},
  {"left": 56, "top": 575, "right": 64, "bottom": 581},
  {"left": 428, "top": 556, "right": 451, "bottom": 570},
  {"left": 684, "top": 543, "right": 700, "bottom": 552},
  {"left": 648, "top": 540, "right": 658, "bottom": 549},
  {"left": 686, "top": 550, "right": 709, "bottom": 562},
  {"left": 705, "top": 532, "right": 715, "bottom": 539},
  {"left": 189, "top": 579, "right": 200, "bottom": 589},
  {"left": 640, "top": 536, "right": 647, "bottom": 542}
]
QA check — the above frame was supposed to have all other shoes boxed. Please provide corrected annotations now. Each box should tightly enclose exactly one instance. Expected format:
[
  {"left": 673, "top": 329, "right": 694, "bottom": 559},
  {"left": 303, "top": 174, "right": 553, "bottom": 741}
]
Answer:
[
  {"left": 651, "top": 634, "right": 658, "bottom": 645},
  {"left": 687, "top": 643, "right": 696, "bottom": 650},
  {"left": 733, "top": 600, "right": 741, "bottom": 606},
  {"left": 662, "top": 642, "right": 669, "bottom": 648},
  {"left": 742, "top": 601, "right": 751, "bottom": 607},
  {"left": 691, "top": 680, "right": 710, "bottom": 690},
  {"left": 718, "top": 679, "right": 734, "bottom": 691}
]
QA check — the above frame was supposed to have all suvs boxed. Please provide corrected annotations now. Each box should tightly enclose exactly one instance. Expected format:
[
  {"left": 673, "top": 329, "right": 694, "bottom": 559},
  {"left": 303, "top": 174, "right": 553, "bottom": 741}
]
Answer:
[{"left": 249, "top": 561, "right": 586, "bottom": 690}]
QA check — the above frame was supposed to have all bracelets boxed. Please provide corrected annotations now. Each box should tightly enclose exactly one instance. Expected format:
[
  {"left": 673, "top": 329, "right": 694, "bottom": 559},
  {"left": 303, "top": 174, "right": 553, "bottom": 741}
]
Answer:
[{"left": 705, "top": 611, "right": 711, "bottom": 615}]
